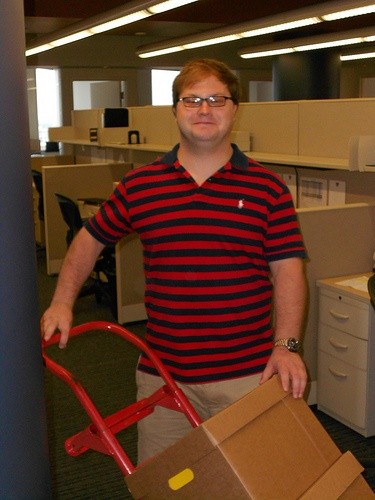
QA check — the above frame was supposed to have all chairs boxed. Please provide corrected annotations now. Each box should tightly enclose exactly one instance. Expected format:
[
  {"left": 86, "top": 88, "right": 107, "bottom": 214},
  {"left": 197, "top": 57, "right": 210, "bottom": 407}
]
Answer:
[
  {"left": 55, "top": 194, "right": 116, "bottom": 304},
  {"left": 32, "top": 170, "right": 44, "bottom": 221}
]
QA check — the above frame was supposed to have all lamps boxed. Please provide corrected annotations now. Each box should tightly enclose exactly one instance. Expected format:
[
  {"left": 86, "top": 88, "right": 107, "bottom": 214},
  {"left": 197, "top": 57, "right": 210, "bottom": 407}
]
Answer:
[
  {"left": 24, "top": 0, "right": 200, "bottom": 58},
  {"left": 134, "top": 1, "right": 375, "bottom": 58},
  {"left": 238, "top": 24, "right": 375, "bottom": 59},
  {"left": 339, "top": 45, "right": 375, "bottom": 62}
]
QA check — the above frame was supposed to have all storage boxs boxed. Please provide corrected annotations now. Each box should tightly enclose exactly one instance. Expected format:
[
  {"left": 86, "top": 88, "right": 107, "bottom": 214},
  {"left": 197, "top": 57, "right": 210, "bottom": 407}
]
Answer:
[{"left": 125, "top": 373, "right": 375, "bottom": 500}]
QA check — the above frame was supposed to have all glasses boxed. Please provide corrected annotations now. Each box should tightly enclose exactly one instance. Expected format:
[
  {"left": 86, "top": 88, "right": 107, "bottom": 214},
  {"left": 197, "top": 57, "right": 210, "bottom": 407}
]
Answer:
[{"left": 176, "top": 96, "right": 232, "bottom": 108}]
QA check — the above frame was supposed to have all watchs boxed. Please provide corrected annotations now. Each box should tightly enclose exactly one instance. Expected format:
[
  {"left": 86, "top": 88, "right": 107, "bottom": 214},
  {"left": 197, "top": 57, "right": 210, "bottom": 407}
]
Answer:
[{"left": 274, "top": 337, "right": 301, "bottom": 353}]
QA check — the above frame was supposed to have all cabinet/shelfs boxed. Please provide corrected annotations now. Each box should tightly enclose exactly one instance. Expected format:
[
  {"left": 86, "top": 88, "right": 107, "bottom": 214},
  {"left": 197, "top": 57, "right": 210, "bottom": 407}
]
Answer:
[{"left": 308, "top": 272, "right": 375, "bottom": 438}]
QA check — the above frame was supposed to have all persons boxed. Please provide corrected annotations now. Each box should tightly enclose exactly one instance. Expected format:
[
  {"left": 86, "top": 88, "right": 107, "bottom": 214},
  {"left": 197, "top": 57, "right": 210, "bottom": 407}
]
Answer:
[{"left": 41, "top": 58, "right": 307, "bottom": 464}]
